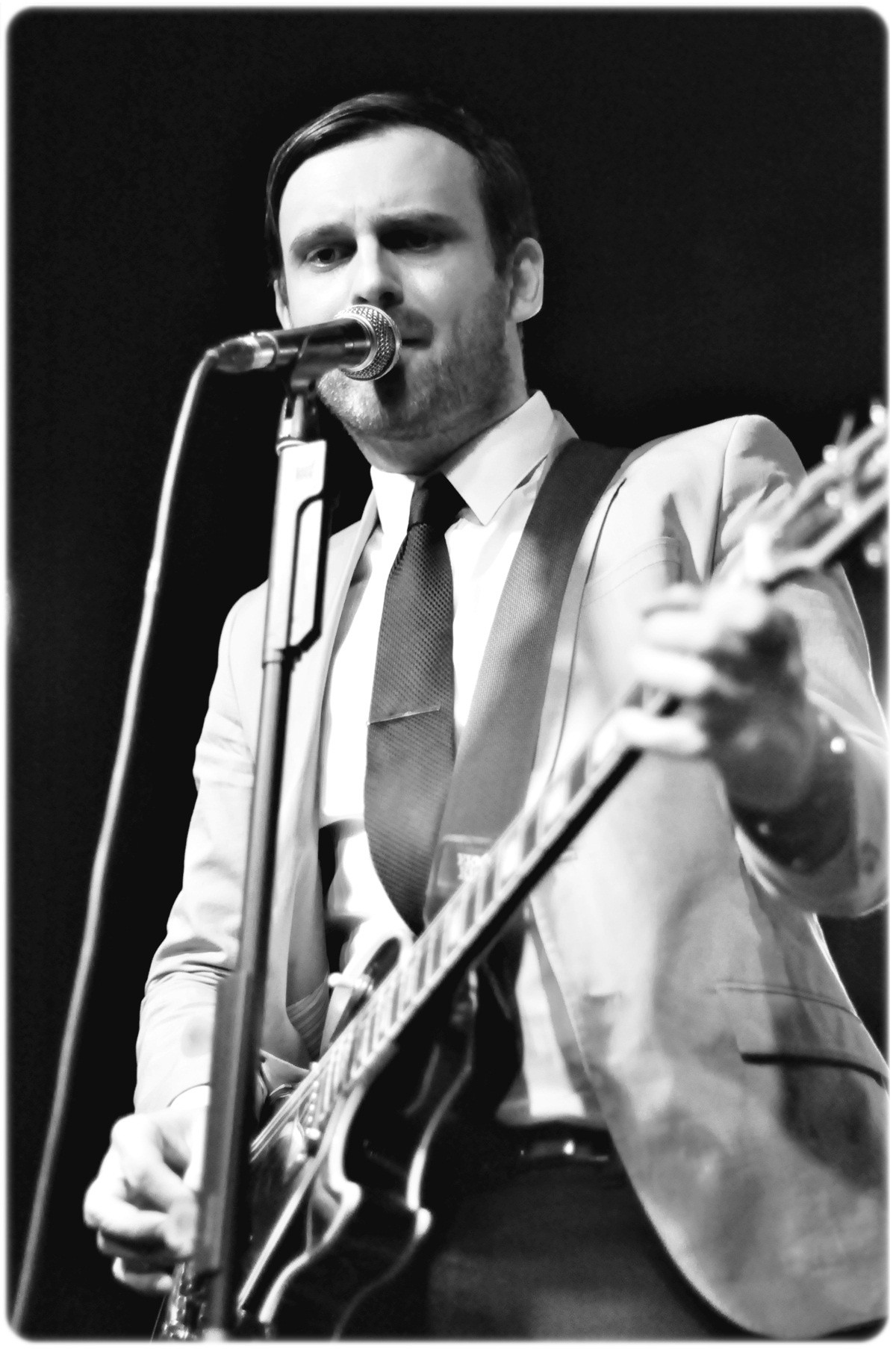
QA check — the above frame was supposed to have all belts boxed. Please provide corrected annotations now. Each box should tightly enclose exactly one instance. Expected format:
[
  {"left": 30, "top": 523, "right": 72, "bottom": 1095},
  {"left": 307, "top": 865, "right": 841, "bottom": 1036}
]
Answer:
[{"left": 449, "top": 1130, "right": 622, "bottom": 1176}]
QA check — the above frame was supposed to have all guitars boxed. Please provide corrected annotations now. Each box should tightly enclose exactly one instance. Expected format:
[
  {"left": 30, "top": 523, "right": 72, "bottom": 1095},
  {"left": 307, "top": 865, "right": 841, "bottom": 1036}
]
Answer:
[{"left": 161, "top": 417, "right": 889, "bottom": 1341}]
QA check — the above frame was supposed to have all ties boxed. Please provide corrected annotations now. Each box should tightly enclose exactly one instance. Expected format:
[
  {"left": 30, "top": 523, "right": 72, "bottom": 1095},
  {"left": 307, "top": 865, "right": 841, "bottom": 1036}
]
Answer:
[{"left": 363, "top": 473, "right": 471, "bottom": 938}]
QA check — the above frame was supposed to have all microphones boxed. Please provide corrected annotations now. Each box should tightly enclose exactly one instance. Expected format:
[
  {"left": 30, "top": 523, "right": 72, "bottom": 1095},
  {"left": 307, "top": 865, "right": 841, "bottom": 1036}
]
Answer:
[{"left": 208, "top": 304, "right": 403, "bottom": 382}]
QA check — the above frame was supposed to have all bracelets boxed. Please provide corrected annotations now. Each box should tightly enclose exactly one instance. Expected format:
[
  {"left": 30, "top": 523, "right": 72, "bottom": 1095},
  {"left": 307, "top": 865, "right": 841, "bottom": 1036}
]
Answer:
[{"left": 728, "top": 752, "right": 852, "bottom": 876}]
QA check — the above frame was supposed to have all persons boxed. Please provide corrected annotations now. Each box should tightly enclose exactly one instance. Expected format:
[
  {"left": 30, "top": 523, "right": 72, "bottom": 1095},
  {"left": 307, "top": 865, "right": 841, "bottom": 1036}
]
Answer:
[{"left": 81, "top": 91, "right": 885, "bottom": 1340}]
